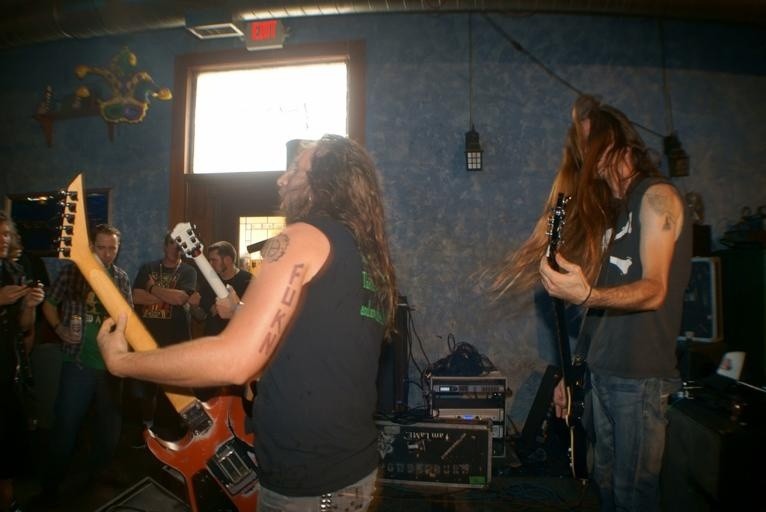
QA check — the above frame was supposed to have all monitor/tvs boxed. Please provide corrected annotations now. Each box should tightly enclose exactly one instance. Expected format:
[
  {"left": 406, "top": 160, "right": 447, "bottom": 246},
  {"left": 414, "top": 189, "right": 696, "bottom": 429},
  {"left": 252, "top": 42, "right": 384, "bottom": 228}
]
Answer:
[{"left": 676, "top": 255, "right": 719, "bottom": 343}]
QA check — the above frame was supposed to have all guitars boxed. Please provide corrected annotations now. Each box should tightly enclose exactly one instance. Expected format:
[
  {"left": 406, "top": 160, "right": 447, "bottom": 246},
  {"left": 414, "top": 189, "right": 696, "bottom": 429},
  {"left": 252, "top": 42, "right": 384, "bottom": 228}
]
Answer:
[
  {"left": 545, "top": 192, "right": 590, "bottom": 486},
  {"left": 170, "top": 221, "right": 243, "bottom": 306},
  {"left": 50, "top": 173, "right": 259, "bottom": 511}
]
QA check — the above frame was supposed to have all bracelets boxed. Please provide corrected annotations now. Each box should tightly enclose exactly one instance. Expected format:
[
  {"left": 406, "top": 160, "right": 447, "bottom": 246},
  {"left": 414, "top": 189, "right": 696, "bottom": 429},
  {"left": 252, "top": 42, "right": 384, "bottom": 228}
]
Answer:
[{"left": 577, "top": 284, "right": 594, "bottom": 308}]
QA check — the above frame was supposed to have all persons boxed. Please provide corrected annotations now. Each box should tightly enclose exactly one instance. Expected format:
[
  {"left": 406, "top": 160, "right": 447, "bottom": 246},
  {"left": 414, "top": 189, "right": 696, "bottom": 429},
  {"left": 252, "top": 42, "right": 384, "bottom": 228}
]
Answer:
[
  {"left": 0, "top": 205, "right": 259, "bottom": 511},
  {"left": 94, "top": 131, "right": 400, "bottom": 512},
  {"left": 486, "top": 92, "right": 695, "bottom": 508}
]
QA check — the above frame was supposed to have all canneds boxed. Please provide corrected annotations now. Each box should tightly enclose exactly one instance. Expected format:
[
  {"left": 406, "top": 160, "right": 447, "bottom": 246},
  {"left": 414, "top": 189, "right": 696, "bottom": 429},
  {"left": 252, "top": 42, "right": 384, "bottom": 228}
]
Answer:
[{"left": 69, "top": 315, "right": 83, "bottom": 340}]
